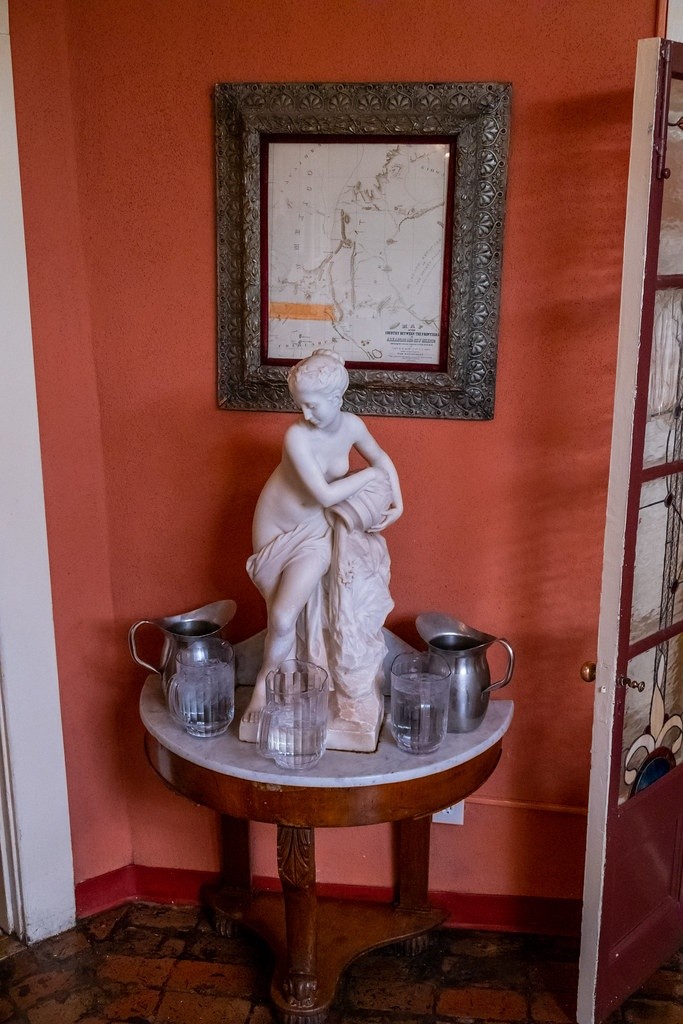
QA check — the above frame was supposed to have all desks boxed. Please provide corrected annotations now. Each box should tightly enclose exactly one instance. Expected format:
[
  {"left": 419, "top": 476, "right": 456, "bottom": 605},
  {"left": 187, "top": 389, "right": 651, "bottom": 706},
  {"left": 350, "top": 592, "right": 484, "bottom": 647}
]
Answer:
[{"left": 139, "top": 671, "right": 513, "bottom": 1024}]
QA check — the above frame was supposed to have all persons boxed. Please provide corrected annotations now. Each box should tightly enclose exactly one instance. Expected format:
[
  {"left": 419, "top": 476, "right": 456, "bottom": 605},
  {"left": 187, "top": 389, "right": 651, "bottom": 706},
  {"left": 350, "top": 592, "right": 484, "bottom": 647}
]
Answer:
[{"left": 234, "top": 346, "right": 409, "bottom": 720}]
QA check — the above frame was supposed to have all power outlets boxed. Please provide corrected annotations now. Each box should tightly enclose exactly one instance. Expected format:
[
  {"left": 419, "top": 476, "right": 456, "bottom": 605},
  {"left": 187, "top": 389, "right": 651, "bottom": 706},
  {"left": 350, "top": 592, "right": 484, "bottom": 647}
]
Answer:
[{"left": 431, "top": 797, "right": 465, "bottom": 826}]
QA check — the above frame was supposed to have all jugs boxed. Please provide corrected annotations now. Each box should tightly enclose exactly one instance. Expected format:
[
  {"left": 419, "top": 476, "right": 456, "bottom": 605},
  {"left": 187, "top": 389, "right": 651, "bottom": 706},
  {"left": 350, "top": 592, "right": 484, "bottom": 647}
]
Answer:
[
  {"left": 164, "top": 635, "right": 240, "bottom": 741},
  {"left": 256, "top": 659, "right": 333, "bottom": 773},
  {"left": 127, "top": 600, "right": 239, "bottom": 709},
  {"left": 414, "top": 611, "right": 514, "bottom": 734},
  {"left": 388, "top": 650, "right": 454, "bottom": 755}
]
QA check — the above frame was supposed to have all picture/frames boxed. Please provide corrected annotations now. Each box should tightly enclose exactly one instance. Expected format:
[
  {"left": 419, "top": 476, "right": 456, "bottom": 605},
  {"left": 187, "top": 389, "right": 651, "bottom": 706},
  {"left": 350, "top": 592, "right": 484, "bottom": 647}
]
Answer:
[{"left": 211, "top": 79, "right": 514, "bottom": 421}]
